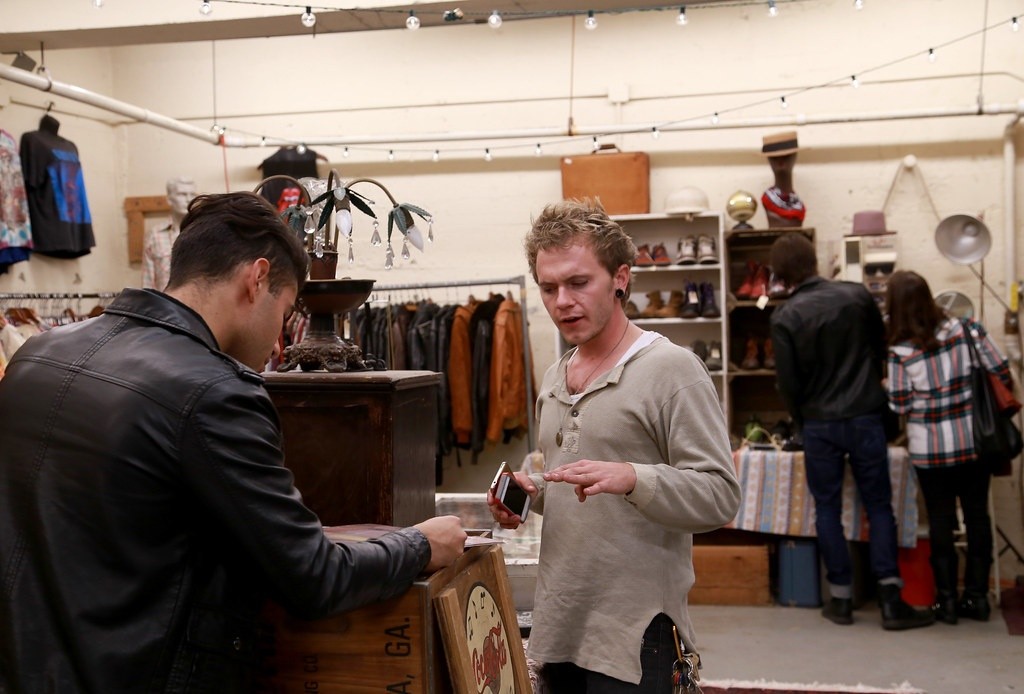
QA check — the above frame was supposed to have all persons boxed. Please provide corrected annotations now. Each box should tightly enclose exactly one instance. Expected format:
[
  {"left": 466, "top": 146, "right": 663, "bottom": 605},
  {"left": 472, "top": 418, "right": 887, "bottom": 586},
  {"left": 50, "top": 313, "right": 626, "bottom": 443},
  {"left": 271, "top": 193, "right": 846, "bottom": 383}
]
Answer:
[
  {"left": 768, "top": 233, "right": 939, "bottom": 631},
  {"left": 141, "top": 178, "right": 198, "bottom": 293},
  {"left": 483, "top": 196, "right": 744, "bottom": 694},
  {"left": 0, "top": 190, "right": 467, "bottom": 694},
  {"left": 882, "top": 268, "right": 1014, "bottom": 626}
]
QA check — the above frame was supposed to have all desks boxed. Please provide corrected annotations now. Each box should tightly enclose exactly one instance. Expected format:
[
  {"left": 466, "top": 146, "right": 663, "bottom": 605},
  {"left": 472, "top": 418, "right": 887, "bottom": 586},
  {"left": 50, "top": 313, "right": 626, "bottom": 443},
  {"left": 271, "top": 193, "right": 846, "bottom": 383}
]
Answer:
[{"left": 717, "top": 444, "right": 922, "bottom": 550}]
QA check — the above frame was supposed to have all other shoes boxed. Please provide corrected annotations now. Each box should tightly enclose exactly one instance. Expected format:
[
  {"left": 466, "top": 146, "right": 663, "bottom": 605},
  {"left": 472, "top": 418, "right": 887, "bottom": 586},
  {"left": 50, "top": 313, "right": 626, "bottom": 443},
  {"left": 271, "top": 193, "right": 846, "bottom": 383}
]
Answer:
[
  {"left": 822, "top": 597, "right": 851, "bottom": 625},
  {"left": 879, "top": 586, "right": 932, "bottom": 628},
  {"left": 959, "top": 591, "right": 990, "bottom": 621},
  {"left": 625, "top": 233, "right": 796, "bottom": 449},
  {"left": 930, "top": 590, "right": 958, "bottom": 625}
]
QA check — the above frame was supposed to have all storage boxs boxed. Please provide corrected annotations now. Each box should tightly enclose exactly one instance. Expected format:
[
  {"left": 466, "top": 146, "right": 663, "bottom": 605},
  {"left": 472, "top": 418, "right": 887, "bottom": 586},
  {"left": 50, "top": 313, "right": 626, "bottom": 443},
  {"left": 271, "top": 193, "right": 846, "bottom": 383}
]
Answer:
[{"left": 686, "top": 540, "right": 820, "bottom": 608}]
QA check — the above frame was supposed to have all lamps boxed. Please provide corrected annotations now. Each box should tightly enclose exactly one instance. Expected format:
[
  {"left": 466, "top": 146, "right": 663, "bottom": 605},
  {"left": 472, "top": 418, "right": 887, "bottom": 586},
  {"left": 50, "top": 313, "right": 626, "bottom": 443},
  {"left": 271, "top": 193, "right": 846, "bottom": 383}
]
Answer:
[
  {"left": 934, "top": 213, "right": 991, "bottom": 331},
  {"left": 250, "top": 166, "right": 435, "bottom": 370}
]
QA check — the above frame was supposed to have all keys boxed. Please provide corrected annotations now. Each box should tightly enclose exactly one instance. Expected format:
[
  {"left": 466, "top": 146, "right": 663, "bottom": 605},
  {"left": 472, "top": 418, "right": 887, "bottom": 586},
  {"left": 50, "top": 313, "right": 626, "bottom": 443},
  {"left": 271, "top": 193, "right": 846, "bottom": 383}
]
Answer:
[{"left": 672, "top": 654, "right": 708, "bottom": 694}]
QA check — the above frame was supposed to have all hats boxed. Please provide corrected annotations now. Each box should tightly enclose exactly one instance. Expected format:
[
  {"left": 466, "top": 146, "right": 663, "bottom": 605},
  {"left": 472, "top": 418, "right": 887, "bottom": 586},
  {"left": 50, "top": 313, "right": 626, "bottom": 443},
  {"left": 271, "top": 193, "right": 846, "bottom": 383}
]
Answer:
[
  {"left": 752, "top": 131, "right": 810, "bottom": 157},
  {"left": 844, "top": 211, "right": 897, "bottom": 237}
]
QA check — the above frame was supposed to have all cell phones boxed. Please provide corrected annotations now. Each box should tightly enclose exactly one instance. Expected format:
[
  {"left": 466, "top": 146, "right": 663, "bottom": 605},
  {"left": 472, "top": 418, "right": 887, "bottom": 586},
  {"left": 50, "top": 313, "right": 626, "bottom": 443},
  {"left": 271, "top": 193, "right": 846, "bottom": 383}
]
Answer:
[{"left": 490, "top": 462, "right": 530, "bottom": 523}]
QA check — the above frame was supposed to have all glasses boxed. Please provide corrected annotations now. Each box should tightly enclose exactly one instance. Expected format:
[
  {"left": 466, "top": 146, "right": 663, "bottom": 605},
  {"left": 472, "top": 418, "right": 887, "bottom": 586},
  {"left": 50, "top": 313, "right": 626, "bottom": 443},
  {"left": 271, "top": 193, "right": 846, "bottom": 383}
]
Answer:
[{"left": 864, "top": 263, "right": 895, "bottom": 276}]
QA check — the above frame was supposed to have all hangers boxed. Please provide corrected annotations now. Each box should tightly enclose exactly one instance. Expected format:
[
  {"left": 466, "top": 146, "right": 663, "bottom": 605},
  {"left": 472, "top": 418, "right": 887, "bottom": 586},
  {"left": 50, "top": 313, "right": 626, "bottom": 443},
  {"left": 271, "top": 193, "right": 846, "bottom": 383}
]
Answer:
[
  {"left": 360, "top": 277, "right": 517, "bottom": 310},
  {"left": 256, "top": 144, "right": 330, "bottom": 170}
]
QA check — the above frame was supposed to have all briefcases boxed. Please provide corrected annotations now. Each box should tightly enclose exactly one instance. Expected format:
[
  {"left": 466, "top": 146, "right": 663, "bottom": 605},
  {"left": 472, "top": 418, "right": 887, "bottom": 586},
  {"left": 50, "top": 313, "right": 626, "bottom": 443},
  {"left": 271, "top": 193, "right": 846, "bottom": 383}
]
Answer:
[
  {"left": 561, "top": 143, "right": 650, "bottom": 215},
  {"left": 779, "top": 541, "right": 822, "bottom": 607}
]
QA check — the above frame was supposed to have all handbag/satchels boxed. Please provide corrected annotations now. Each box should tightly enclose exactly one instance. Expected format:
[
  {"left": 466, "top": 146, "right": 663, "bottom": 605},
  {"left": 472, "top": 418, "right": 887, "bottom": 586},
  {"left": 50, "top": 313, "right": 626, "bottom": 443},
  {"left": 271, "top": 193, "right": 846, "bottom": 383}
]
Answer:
[{"left": 961, "top": 321, "right": 1024, "bottom": 476}]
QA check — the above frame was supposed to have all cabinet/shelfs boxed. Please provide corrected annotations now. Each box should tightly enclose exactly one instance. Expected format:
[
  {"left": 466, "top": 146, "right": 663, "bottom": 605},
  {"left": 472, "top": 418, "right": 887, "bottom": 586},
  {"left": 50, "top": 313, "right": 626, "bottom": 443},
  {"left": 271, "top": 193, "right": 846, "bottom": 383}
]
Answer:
[{"left": 553, "top": 209, "right": 815, "bottom": 450}]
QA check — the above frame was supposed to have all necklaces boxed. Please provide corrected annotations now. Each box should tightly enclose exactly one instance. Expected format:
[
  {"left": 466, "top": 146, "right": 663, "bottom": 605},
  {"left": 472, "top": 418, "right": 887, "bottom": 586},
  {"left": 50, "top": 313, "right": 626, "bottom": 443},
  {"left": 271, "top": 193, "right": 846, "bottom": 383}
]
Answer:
[{"left": 556, "top": 319, "right": 630, "bottom": 446}]
